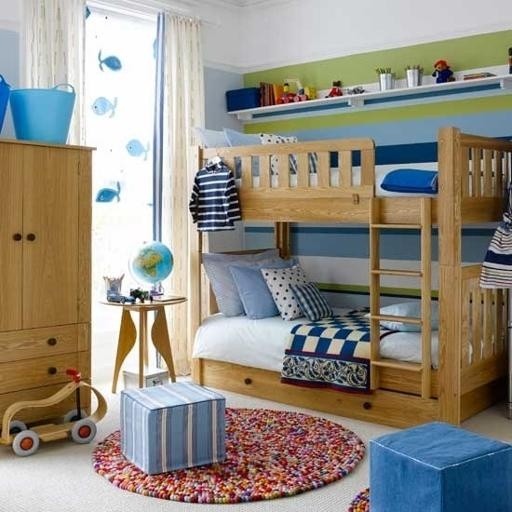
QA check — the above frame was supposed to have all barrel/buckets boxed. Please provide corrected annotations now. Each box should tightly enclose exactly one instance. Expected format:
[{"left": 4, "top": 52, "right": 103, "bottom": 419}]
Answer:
[
  {"left": 11, "top": 82, "right": 77, "bottom": 145},
  {"left": 0, "top": 74, "right": 11, "bottom": 138}
]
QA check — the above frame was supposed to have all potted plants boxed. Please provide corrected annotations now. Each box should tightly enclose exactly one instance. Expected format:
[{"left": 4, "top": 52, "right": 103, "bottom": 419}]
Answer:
[
  {"left": 404, "top": 64, "right": 424, "bottom": 87},
  {"left": 375, "top": 65, "right": 394, "bottom": 91}
]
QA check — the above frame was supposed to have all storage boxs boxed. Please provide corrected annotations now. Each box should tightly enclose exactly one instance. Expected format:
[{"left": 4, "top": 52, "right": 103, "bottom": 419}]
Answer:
[{"left": 121, "top": 363, "right": 169, "bottom": 392}]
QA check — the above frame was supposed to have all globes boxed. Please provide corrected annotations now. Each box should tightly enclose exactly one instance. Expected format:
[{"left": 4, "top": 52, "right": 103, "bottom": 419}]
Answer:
[{"left": 128, "top": 241, "right": 174, "bottom": 296}]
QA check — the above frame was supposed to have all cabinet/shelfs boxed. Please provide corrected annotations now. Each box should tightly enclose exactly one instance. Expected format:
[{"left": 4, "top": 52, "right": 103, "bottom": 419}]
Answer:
[{"left": 0, "top": 138, "right": 92, "bottom": 449}]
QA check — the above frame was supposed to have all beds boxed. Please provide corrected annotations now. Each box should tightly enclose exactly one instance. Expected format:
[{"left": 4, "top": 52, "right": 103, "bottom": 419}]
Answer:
[{"left": 184, "top": 120, "right": 511, "bottom": 431}]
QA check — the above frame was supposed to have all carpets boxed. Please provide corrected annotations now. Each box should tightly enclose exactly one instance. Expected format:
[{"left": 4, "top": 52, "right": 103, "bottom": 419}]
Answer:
[
  {"left": 92, "top": 409, "right": 365, "bottom": 503},
  {"left": 349, "top": 487, "right": 371, "bottom": 511}
]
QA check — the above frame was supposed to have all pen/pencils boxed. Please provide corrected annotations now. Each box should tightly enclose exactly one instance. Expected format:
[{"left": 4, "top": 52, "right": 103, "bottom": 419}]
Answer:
[
  {"left": 375, "top": 64, "right": 391, "bottom": 74},
  {"left": 404, "top": 64, "right": 424, "bottom": 71},
  {"left": 103, "top": 273, "right": 124, "bottom": 288}
]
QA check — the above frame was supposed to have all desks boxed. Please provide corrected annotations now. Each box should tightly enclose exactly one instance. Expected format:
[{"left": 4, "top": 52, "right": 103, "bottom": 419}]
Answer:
[{"left": 96, "top": 292, "right": 189, "bottom": 392}]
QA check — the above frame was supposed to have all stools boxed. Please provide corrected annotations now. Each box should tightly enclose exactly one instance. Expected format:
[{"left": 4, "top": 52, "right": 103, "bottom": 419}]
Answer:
[
  {"left": 121, "top": 379, "right": 226, "bottom": 477},
  {"left": 369, "top": 420, "right": 512, "bottom": 511}
]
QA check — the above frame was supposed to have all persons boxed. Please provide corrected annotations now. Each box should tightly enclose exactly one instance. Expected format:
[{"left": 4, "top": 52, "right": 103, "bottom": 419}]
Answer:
[{"left": 430, "top": 58, "right": 456, "bottom": 84}]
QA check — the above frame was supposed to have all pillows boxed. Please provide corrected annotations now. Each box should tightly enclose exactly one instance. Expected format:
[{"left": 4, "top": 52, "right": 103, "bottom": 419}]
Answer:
[
  {"left": 194, "top": 125, "right": 230, "bottom": 173},
  {"left": 289, "top": 140, "right": 332, "bottom": 173},
  {"left": 260, "top": 263, "right": 309, "bottom": 320},
  {"left": 365, "top": 302, "right": 438, "bottom": 332},
  {"left": 196, "top": 249, "right": 282, "bottom": 319},
  {"left": 227, "top": 257, "right": 300, "bottom": 321},
  {"left": 290, "top": 281, "right": 333, "bottom": 322},
  {"left": 261, "top": 133, "right": 301, "bottom": 175},
  {"left": 225, "top": 127, "right": 265, "bottom": 176}
]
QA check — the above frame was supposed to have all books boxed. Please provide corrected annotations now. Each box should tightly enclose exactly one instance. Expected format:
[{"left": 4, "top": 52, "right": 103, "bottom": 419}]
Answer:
[{"left": 260, "top": 82, "right": 282, "bottom": 106}]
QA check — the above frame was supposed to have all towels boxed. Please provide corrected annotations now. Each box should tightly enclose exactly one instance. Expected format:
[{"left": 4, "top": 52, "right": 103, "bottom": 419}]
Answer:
[{"left": 380, "top": 168, "right": 438, "bottom": 194}]
